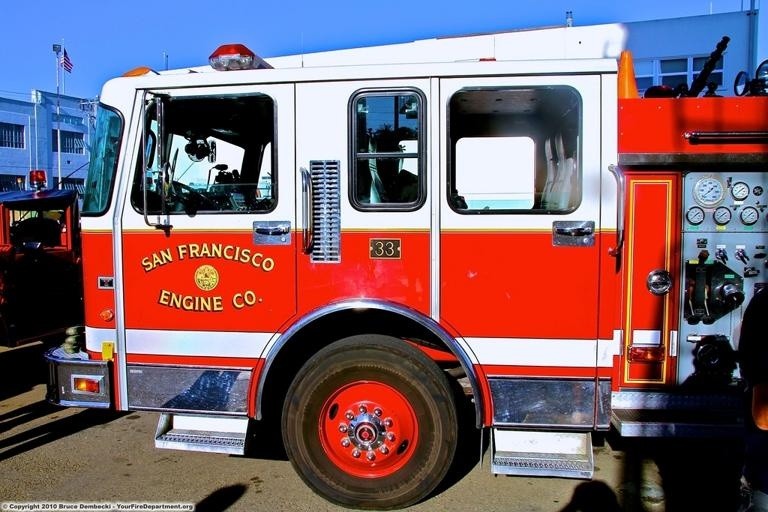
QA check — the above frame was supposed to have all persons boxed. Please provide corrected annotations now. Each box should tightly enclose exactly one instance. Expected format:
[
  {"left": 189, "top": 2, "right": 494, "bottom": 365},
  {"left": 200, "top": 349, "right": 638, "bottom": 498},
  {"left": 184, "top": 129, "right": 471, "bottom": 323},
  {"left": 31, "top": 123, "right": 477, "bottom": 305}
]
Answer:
[{"left": 739, "top": 286, "right": 768, "bottom": 512}]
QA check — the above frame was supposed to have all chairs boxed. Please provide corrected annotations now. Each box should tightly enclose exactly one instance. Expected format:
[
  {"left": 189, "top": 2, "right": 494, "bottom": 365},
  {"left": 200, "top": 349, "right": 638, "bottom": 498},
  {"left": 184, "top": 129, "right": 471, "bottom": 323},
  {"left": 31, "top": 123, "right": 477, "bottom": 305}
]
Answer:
[{"left": 540, "top": 131, "right": 579, "bottom": 210}]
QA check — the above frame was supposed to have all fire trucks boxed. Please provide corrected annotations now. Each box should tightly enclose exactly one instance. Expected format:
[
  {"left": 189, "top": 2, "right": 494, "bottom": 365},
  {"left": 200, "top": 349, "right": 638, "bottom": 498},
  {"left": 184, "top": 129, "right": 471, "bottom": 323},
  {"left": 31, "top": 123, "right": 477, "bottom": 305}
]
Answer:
[{"left": 0, "top": 10, "right": 768, "bottom": 511}]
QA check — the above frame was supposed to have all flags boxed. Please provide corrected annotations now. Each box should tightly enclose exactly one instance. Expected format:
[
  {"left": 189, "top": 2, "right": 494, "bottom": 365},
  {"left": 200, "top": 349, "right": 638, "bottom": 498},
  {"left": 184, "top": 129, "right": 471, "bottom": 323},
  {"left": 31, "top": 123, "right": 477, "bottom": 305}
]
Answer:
[{"left": 61, "top": 48, "right": 73, "bottom": 73}]
